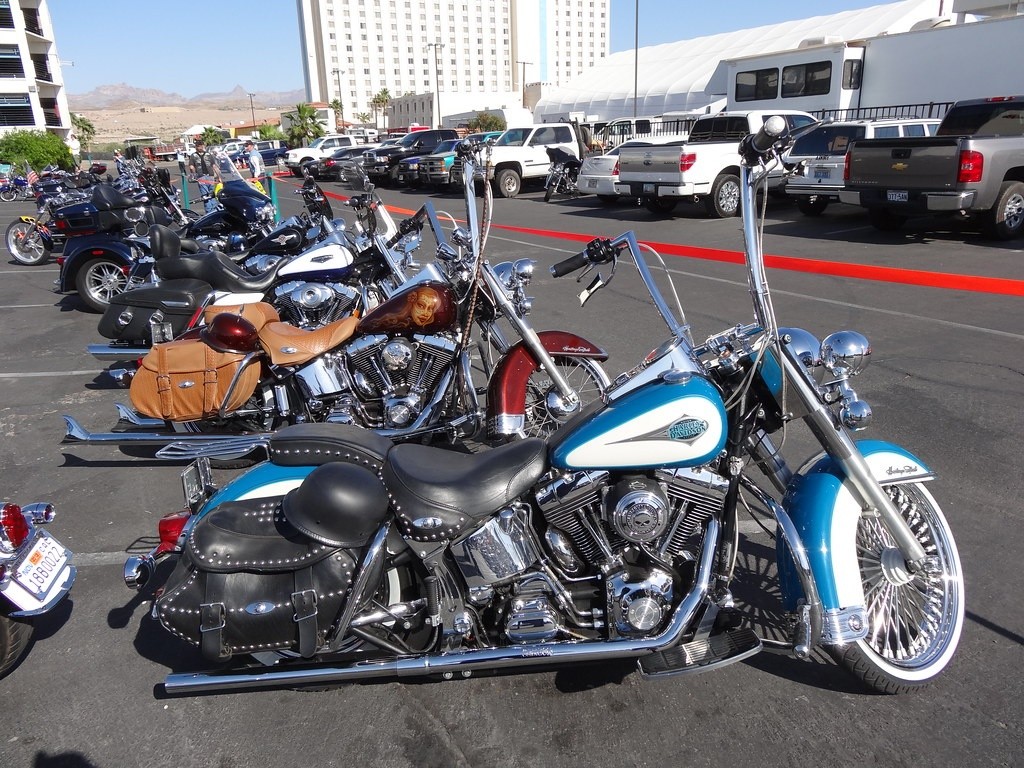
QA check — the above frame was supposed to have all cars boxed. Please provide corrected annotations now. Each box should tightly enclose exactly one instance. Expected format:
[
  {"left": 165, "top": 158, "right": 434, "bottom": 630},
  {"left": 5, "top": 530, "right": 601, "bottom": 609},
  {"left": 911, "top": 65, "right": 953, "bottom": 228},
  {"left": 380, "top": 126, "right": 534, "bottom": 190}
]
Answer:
[
  {"left": 783, "top": 117, "right": 943, "bottom": 217},
  {"left": 581, "top": 116, "right": 662, "bottom": 147},
  {"left": 209, "top": 136, "right": 288, "bottom": 165},
  {"left": 343, "top": 137, "right": 402, "bottom": 182},
  {"left": 303, "top": 158, "right": 318, "bottom": 177},
  {"left": 576, "top": 136, "right": 688, "bottom": 203},
  {"left": 318, "top": 146, "right": 378, "bottom": 182}
]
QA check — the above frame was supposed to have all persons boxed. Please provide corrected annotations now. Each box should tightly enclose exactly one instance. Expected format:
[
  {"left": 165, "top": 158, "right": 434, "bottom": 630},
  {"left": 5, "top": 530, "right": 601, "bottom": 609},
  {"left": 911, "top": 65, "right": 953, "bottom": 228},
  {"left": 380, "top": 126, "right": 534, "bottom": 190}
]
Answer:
[
  {"left": 69, "top": 134, "right": 81, "bottom": 170},
  {"left": 113, "top": 149, "right": 125, "bottom": 175},
  {"left": 756, "top": 74, "right": 769, "bottom": 97},
  {"left": 805, "top": 73, "right": 823, "bottom": 92},
  {"left": 244, "top": 140, "right": 266, "bottom": 190},
  {"left": 189, "top": 140, "right": 219, "bottom": 214},
  {"left": 212, "top": 151, "right": 225, "bottom": 179},
  {"left": 177, "top": 148, "right": 186, "bottom": 175}
]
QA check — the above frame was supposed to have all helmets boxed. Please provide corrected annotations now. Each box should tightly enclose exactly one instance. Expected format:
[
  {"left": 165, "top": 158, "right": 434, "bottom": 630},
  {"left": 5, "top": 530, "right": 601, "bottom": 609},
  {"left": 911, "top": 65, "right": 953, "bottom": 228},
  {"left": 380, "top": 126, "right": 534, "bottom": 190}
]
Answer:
[
  {"left": 199, "top": 312, "right": 258, "bottom": 354},
  {"left": 282, "top": 462, "right": 389, "bottom": 548}
]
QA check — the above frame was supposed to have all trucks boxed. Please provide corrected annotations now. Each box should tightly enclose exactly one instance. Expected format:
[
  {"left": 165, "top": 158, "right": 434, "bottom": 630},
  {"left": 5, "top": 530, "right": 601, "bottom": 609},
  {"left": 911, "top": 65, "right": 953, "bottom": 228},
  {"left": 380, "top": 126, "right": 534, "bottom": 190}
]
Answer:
[
  {"left": 452, "top": 117, "right": 616, "bottom": 198},
  {"left": 155, "top": 142, "right": 197, "bottom": 162}
]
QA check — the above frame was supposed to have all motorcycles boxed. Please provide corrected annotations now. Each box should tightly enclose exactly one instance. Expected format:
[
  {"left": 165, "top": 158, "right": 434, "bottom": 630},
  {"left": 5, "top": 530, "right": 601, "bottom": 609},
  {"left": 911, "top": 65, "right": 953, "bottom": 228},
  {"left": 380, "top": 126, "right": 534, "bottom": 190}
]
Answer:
[
  {"left": 62, "top": 135, "right": 616, "bottom": 471},
  {"left": 0, "top": 162, "right": 433, "bottom": 389},
  {"left": 0, "top": 115, "right": 968, "bottom": 694},
  {"left": 544, "top": 145, "right": 584, "bottom": 202},
  {"left": 0, "top": 500, "right": 77, "bottom": 680}
]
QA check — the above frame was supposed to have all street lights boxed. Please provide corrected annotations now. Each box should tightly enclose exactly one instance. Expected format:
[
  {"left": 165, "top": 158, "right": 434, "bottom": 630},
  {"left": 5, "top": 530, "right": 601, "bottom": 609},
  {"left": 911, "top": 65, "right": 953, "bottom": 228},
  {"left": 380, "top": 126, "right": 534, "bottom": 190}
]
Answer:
[
  {"left": 245, "top": 93, "right": 257, "bottom": 139},
  {"left": 330, "top": 42, "right": 533, "bottom": 134}
]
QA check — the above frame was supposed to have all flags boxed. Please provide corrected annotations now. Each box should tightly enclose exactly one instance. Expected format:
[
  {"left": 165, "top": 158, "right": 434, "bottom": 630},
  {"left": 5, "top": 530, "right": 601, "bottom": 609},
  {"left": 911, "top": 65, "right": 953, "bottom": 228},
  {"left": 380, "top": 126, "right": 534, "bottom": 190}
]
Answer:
[{"left": 26, "top": 164, "right": 39, "bottom": 185}]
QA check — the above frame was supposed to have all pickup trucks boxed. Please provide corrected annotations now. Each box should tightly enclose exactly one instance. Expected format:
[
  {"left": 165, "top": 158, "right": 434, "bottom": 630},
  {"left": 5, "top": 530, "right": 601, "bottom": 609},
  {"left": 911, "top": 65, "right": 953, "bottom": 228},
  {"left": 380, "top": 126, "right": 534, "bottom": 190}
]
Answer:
[
  {"left": 284, "top": 135, "right": 383, "bottom": 176},
  {"left": 614, "top": 110, "right": 824, "bottom": 218},
  {"left": 362, "top": 128, "right": 524, "bottom": 191},
  {"left": 838, "top": 96, "right": 1024, "bottom": 244}
]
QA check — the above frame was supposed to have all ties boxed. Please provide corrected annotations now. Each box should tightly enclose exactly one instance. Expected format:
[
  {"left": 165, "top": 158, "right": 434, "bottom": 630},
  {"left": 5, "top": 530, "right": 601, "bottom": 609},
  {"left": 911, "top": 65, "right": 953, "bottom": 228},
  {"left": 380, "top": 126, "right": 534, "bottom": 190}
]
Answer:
[{"left": 195, "top": 151, "right": 209, "bottom": 175}]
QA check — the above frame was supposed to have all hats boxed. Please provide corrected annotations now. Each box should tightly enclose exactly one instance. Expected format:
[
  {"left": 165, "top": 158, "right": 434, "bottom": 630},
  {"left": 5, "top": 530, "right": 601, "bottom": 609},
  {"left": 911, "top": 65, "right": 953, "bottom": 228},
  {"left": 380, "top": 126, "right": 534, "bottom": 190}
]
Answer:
[{"left": 245, "top": 140, "right": 254, "bottom": 145}]
